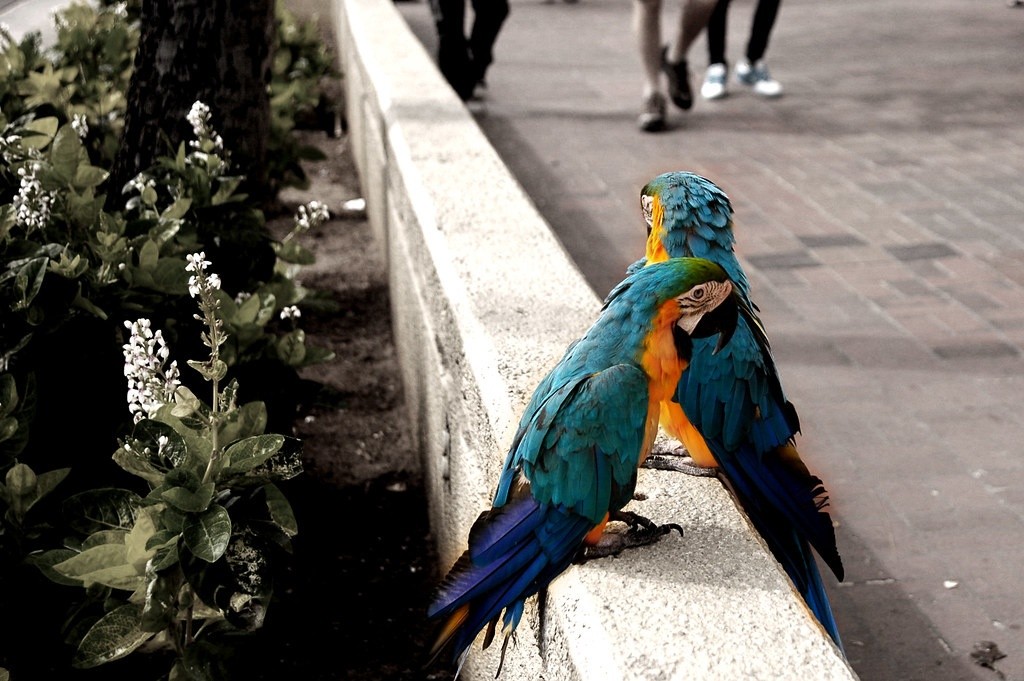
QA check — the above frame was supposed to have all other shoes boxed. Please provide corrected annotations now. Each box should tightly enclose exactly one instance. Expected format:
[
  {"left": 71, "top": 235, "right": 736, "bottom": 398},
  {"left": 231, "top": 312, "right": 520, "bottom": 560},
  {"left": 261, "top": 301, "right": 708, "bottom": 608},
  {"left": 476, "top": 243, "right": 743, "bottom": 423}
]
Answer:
[
  {"left": 735, "top": 59, "right": 783, "bottom": 96},
  {"left": 636, "top": 92, "right": 666, "bottom": 130},
  {"left": 659, "top": 47, "right": 693, "bottom": 110},
  {"left": 700, "top": 62, "right": 726, "bottom": 98}
]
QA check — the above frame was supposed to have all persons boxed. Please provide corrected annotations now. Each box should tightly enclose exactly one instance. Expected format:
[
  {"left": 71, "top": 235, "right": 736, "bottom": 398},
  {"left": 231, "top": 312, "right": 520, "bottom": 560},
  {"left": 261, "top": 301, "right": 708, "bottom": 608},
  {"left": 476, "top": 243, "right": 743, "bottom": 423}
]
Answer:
[
  {"left": 700, "top": 0, "right": 784, "bottom": 101},
  {"left": 426, "top": 0, "right": 511, "bottom": 114},
  {"left": 624, "top": 0, "right": 717, "bottom": 134}
]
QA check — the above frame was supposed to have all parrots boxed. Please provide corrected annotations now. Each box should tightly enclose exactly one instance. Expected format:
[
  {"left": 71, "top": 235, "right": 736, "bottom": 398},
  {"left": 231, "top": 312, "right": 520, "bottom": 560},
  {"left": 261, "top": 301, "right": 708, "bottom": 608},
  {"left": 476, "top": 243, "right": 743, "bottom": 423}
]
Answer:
[
  {"left": 421, "top": 255, "right": 742, "bottom": 680},
  {"left": 639, "top": 167, "right": 849, "bottom": 659}
]
